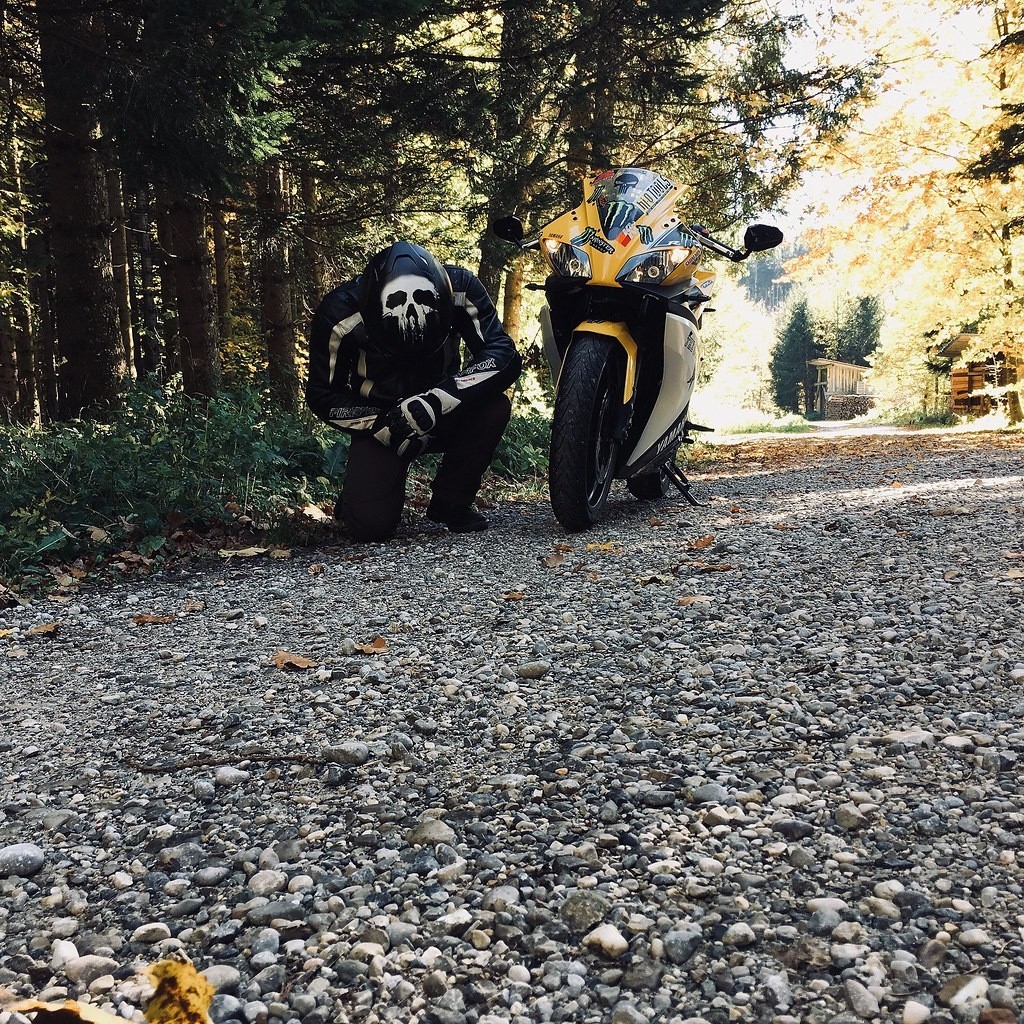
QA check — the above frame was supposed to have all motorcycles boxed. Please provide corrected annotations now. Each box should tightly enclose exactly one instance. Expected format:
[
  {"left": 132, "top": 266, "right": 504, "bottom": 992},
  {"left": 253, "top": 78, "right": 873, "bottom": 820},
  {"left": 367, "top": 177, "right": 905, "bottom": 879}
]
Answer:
[{"left": 519, "top": 167, "right": 784, "bottom": 531}]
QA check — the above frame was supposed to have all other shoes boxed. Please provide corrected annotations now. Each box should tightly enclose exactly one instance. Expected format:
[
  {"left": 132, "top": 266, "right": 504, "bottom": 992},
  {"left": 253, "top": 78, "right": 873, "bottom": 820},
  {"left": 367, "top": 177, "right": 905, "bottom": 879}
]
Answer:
[
  {"left": 334, "top": 488, "right": 346, "bottom": 520},
  {"left": 425, "top": 499, "right": 487, "bottom": 533}
]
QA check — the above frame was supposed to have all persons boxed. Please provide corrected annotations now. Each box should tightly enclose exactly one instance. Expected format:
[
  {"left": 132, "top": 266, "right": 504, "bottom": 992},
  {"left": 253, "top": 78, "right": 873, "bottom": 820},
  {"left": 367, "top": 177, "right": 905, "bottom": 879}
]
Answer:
[{"left": 306, "top": 241, "right": 521, "bottom": 542}]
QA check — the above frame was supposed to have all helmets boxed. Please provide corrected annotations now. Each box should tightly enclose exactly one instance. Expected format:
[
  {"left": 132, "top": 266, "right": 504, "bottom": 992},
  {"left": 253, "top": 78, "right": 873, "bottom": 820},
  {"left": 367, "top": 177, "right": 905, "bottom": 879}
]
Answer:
[{"left": 357, "top": 241, "right": 453, "bottom": 363}]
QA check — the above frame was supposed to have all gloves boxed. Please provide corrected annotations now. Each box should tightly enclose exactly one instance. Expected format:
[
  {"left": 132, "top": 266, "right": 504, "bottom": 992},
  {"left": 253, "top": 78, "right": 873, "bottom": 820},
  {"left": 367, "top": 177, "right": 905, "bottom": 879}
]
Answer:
[
  {"left": 369, "top": 398, "right": 439, "bottom": 459},
  {"left": 387, "top": 386, "right": 462, "bottom": 450}
]
